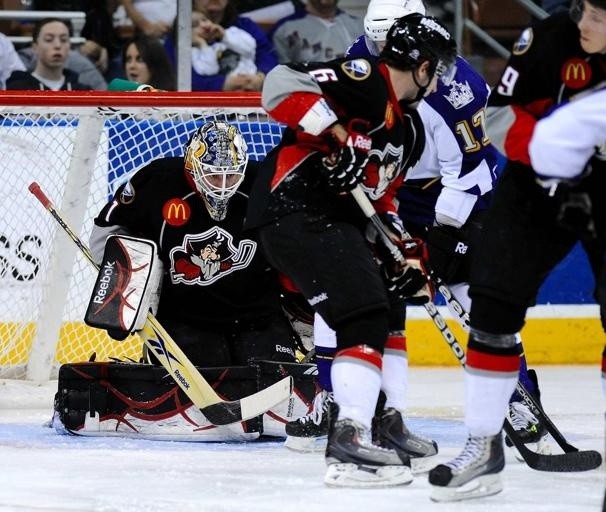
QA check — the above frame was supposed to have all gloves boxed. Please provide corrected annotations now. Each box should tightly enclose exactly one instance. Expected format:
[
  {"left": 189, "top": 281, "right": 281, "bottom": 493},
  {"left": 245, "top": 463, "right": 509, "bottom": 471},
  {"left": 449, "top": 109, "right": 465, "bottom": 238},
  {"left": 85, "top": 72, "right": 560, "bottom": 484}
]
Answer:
[
  {"left": 365, "top": 209, "right": 416, "bottom": 270},
  {"left": 321, "top": 134, "right": 372, "bottom": 197},
  {"left": 383, "top": 236, "right": 434, "bottom": 306},
  {"left": 429, "top": 223, "right": 470, "bottom": 289}
]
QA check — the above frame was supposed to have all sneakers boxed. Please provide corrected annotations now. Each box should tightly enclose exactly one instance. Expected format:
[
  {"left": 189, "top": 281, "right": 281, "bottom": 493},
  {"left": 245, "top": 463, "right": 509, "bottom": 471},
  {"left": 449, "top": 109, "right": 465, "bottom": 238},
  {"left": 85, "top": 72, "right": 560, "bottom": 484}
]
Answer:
[
  {"left": 504, "top": 368, "right": 553, "bottom": 464},
  {"left": 369, "top": 389, "right": 441, "bottom": 475},
  {"left": 284, "top": 389, "right": 339, "bottom": 454},
  {"left": 322, "top": 414, "right": 414, "bottom": 490},
  {"left": 426, "top": 429, "right": 506, "bottom": 502}
]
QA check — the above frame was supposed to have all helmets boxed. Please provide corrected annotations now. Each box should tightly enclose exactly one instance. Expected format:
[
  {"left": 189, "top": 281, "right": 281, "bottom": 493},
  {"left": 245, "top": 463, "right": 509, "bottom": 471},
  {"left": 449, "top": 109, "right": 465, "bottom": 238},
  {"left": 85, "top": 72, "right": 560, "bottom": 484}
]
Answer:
[
  {"left": 381, "top": 11, "right": 456, "bottom": 70},
  {"left": 184, "top": 120, "right": 249, "bottom": 221},
  {"left": 361, "top": 0, "right": 427, "bottom": 44}
]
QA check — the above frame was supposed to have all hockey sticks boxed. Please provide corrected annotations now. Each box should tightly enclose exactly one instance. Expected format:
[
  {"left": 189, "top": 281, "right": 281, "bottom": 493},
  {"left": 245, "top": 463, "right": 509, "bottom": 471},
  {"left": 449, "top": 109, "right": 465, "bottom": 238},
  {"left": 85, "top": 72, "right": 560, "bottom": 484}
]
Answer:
[
  {"left": 421, "top": 263, "right": 579, "bottom": 453},
  {"left": 328, "top": 149, "right": 602, "bottom": 473},
  {"left": 27, "top": 180, "right": 295, "bottom": 427}
]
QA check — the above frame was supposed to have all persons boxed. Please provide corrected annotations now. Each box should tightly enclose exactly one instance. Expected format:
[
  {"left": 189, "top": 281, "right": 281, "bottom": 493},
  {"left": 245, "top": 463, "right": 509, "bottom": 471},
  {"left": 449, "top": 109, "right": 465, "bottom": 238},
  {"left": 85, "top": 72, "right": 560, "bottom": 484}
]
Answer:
[
  {"left": 285, "top": 0, "right": 541, "bottom": 463},
  {"left": 48, "top": 120, "right": 307, "bottom": 444},
  {"left": 243, "top": 14, "right": 444, "bottom": 490},
  {"left": 0, "top": 0, "right": 365, "bottom": 93},
  {"left": 429, "top": 1, "right": 606, "bottom": 511}
]
